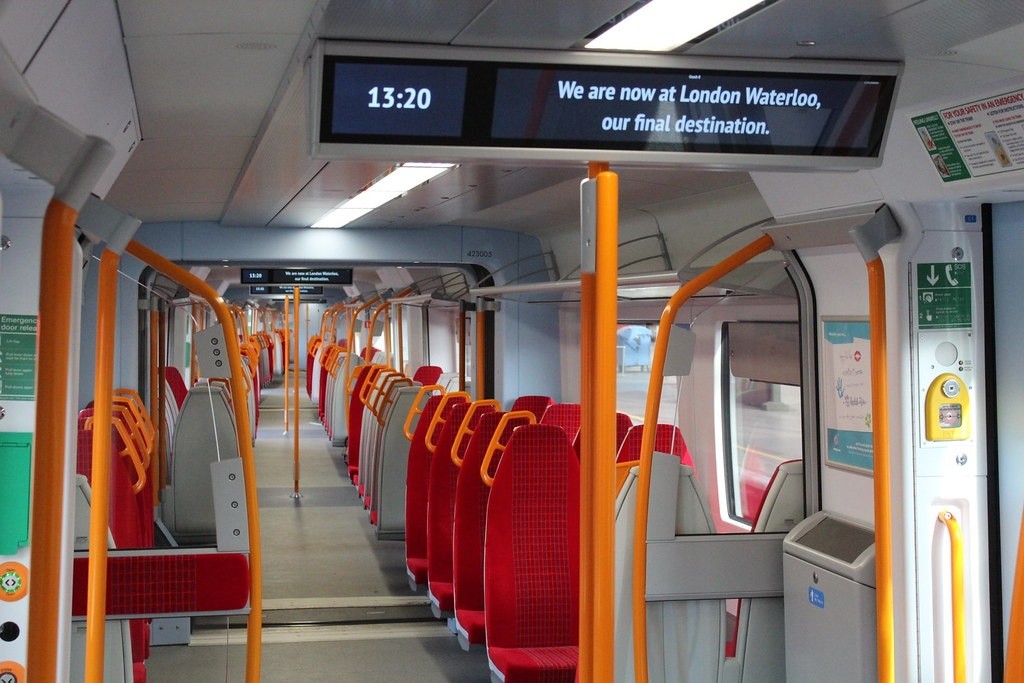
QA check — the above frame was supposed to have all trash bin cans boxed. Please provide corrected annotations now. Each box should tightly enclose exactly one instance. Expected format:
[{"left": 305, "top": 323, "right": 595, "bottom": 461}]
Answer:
[{"left": 781, "top": 510, "right": 878, "bottom": 682}]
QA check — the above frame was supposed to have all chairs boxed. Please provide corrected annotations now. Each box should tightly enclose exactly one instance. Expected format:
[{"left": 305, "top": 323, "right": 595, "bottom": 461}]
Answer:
[
  {"left": 69, "top": 329, "right": 285, "bottom": 683},
  {"left": 307, "top": 335, "right": 805, "bottom": 683}
]
